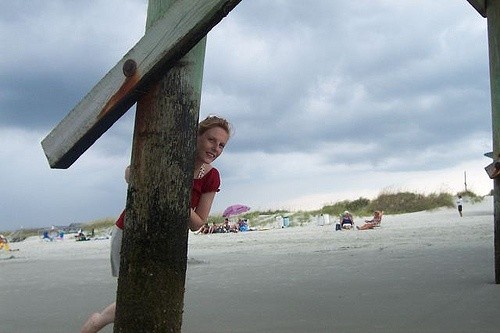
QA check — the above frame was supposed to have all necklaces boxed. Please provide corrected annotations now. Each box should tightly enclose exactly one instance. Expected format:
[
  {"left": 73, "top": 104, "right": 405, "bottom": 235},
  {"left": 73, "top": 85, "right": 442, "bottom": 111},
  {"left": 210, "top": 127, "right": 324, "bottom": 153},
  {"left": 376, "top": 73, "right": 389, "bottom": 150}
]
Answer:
[{"left": 197, "top": 163, "right": 205, "bottom": 179}]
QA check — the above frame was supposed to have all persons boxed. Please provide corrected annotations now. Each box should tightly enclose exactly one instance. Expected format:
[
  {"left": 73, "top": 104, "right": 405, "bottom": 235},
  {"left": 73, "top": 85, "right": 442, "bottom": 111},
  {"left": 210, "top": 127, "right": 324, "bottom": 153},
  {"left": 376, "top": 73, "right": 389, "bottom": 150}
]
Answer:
[
  {"left": 194, "top": 223, "right": 209, "bottom": 235},
  {"left": 208, "top": 222, "right": 217, "bottom": 234},
  {"left": 74, "top": 227, "right": 96, "bottom": 241},
  {"left": 0, "top": 235, "right": 11, "bottom": 252},
  {"left": 356, "top": 211, "right": 381, "bottom": 230},
  {"left": 456, "top": 195, "right": 463, "bottom": 217},
  {"left": 80, "top": 116, "right": 230, "bottom": 333},
  {"left": 223, "top": 218, "right": 231, "bottom": 233},
  {"left": 41, "top": 225, "right": 64, "bottom": 241},
  {"left": 236, "top": 219, "right": 247, "bottom": 232},
  {"left": 341, "top": 211, "right": 352, "bottom": 226}
]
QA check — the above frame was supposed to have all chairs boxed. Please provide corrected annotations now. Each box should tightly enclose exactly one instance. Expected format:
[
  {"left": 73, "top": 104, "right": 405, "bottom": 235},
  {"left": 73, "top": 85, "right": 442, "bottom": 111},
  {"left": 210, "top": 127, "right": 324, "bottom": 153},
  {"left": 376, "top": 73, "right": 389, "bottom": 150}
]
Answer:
[{"left": 339, "top": 212, "right": 382, "bottom": 235}]
